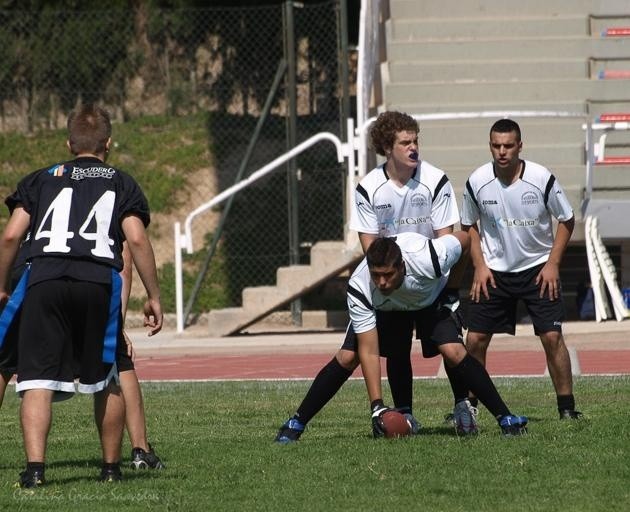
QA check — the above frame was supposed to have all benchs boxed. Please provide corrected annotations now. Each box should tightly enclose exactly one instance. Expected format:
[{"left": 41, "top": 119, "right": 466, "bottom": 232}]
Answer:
[{"left": 581, "top": 12, "right": 630, "bottom": 201}]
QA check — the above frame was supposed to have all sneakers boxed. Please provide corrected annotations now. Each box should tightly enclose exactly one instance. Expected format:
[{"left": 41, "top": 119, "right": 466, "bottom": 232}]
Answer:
[
  {"left": 272, "top": 418, "right": 305, "bottom": 442},
  {"left": 501, "top": 415, "right": 528, "bottom": 429},
  {"left": 17, "top": 470, "right": 46, "bottom": 489},
  {"left": 131, "top": 446, "right": 166, "bottom": 470},
  {"left": 454, "top": 400, "right": 478, "bottom": 438},
  {"left": 101, "top": 466, "right": 122, "bottom": 482},
  {"left": 402, "top": 414, "right": 424, "bottom": 434}
]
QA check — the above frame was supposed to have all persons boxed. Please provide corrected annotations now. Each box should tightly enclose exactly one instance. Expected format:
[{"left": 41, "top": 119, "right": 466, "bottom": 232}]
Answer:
[
  {"left": 0, "top": 105, "right": 163, "bottom": 491},
  {"left": 355, "top": 111, "right": 479, "bottom": 437},
  {"left": 443, "top": 119, "right": 583, "bottom": 422},
  {"left": 0, "top": 232, "right": 168, "bottom": 472},
  {"left": 274, "top": 230, "right": 530, "bottom": 443}
]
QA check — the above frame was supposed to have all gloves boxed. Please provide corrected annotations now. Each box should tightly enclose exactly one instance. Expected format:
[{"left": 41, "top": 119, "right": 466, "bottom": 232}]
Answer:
[{"left": 371, "top": 398, "right": 410, "bottom": 438}]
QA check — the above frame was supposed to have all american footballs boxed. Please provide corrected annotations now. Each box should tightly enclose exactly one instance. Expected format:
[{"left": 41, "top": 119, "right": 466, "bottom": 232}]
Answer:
[{"left": 381, "top": 411, "right": 410, "bottom": 438}]
